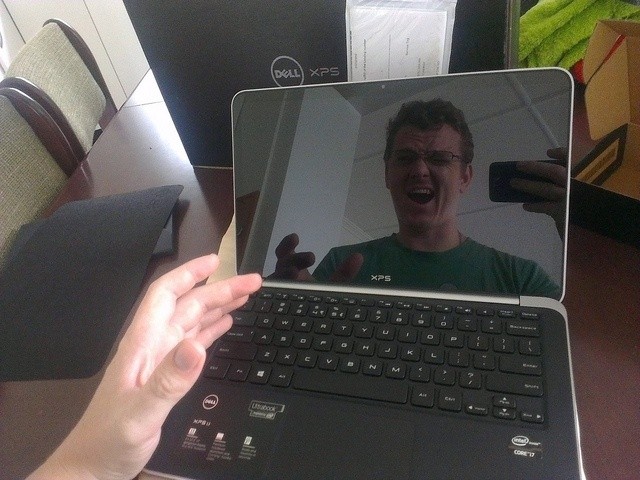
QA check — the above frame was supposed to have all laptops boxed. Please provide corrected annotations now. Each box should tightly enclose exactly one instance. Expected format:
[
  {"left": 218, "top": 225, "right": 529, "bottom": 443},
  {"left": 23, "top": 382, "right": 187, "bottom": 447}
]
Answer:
[
  {"left": 142, "top": 67, "right": 587, "bottom": 480},
  {"left": 122, "top": 0, "right": 522, "bottom": 170}
]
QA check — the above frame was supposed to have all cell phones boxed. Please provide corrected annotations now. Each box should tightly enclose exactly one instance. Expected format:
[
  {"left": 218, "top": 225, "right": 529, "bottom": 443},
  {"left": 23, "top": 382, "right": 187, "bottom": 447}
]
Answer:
[{"left": 490, "top": 162, "right": 565, "bottom": 202}]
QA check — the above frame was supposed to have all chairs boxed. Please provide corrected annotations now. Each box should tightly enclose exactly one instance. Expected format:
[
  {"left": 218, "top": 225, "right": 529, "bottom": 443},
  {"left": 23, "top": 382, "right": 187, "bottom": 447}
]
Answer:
[
  {"left": 1, "top": 16, "right": 119, "bottom": 161},
  {"left": 1, "top": 86, "right": 83, "bottom": 267}
]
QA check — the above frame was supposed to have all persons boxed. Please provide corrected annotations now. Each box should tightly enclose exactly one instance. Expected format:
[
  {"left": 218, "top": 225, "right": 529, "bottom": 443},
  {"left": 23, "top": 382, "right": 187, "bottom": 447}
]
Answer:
[
  {"left": 274, "top": 96, "right": 568, "bottom": 302},
  {"left": 26, "top": 253, "right": 264, "bottom": 480}
]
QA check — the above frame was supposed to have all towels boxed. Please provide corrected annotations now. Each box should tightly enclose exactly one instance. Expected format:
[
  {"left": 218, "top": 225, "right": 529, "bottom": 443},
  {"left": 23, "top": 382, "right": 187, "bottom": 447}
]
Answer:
[{"left": 517, "top": 0, "right": 640, "bottom": 72}]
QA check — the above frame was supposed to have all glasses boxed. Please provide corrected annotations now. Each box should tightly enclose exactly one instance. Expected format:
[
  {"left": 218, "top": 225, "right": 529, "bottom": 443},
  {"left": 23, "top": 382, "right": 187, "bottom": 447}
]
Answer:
[{"left": 387, "top": 150, "right": 461, "bottom": 165}]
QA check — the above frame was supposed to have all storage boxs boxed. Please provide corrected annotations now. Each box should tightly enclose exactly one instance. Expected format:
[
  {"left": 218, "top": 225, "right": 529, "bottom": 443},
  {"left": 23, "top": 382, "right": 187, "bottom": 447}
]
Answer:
[{"left": 569, "top": 19, "right": 640, "bottom": 246}]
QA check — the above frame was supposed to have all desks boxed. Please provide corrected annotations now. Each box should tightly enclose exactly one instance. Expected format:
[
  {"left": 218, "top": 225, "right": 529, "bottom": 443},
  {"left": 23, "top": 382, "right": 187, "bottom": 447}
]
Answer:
[{"left": 0, "top": 69, "right": 640, "bottom": 479}]
